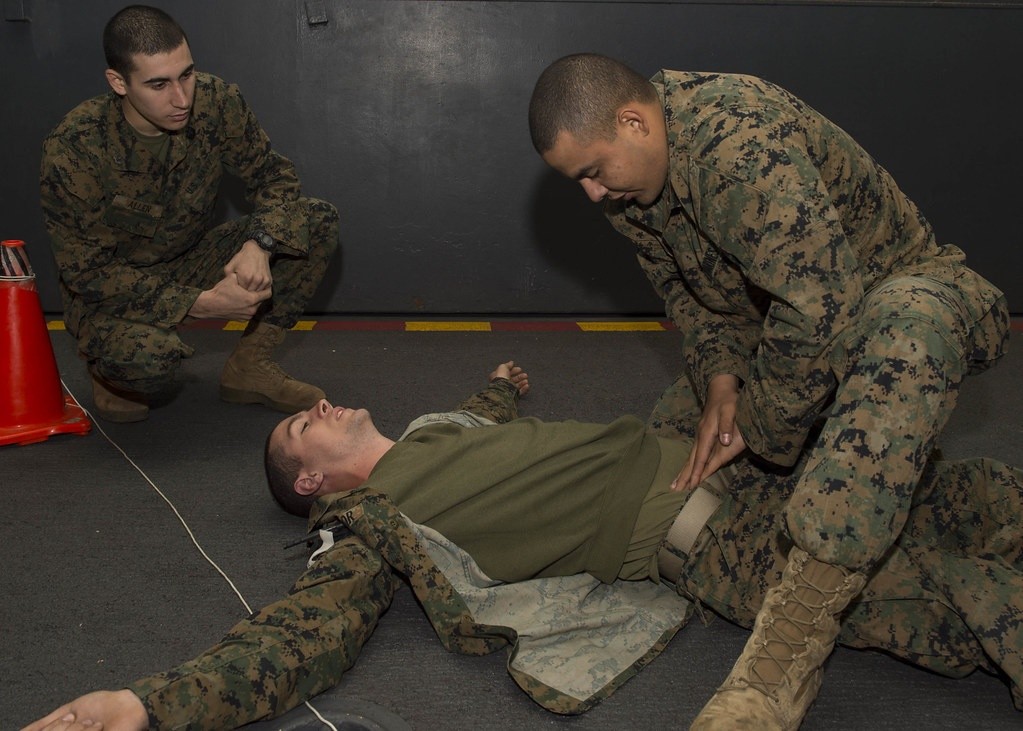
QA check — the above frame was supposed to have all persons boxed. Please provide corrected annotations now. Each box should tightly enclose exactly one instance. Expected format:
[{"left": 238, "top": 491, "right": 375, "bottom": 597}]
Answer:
[
  {"left": 528, "top": 51, "right": 1012, "bottom": 731},
  {"left": 40, "top": 7, "right": 343, "bottom": 422},
  {"left": 23, "top": 359, "right": 1023, "bottom": 731}
]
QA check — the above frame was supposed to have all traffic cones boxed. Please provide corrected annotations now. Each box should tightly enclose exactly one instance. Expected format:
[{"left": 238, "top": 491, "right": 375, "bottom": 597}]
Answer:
[{"left": 0, "top": 240, "right": 92, "bottom": 447}]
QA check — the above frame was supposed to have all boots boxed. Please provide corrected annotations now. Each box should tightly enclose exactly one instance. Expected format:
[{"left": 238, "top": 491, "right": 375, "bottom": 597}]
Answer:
[
  {"left": 689, "top": 546, "right": 869, "bottom": 731},
  {"left": 221, "top": 319, "right": 326, "bottom": 414},
  {"left": 92, "top": 376, "right": 151, "bottom": 422}
]
